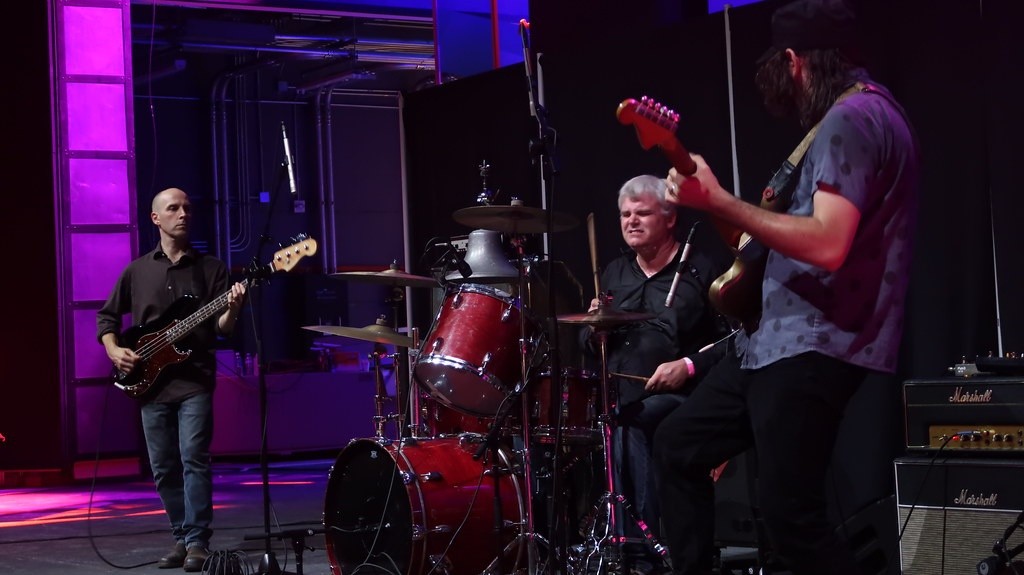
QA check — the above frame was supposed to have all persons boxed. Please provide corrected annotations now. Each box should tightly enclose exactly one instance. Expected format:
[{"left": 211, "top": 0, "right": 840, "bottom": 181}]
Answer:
[
  {"left": 647, "top": 1, "right": 924, "bottom": 575},
  {"left": 580, "top": 174, "right": 734, "bottom": 575},
  {"left": 94, "top": 187, "right": 247, "bottom": 572}
]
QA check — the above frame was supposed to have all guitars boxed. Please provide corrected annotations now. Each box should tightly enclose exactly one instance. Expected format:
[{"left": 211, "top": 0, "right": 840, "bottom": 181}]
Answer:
[
  {"left": 614, "top": 91, "right": 776, "bottom": 318},
  {"left": 110, "top": 232, "right": 317, "bottom": 399}
]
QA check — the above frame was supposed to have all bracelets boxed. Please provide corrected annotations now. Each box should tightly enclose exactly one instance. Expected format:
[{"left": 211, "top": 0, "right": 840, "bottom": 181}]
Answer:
[{"left": 682, "top": 357, "right": 695, "bottom": 378}]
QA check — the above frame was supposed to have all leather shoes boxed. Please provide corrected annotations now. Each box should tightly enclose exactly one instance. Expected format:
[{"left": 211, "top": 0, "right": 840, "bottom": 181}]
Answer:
[
  {"left": 158, "top": 543, "right": 187, "bottom": 567},
  {"left": 183, "top": 547, "right": 206, "bottom": 570}
]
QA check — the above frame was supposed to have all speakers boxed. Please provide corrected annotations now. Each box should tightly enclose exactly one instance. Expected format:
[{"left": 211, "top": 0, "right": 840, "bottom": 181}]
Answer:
[{"left": 892, "top": 451, "right": 1024, "bottom": 575}]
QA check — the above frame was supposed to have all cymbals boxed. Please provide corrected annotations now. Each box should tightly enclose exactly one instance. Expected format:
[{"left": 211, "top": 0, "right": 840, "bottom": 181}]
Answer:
[
  {"left": 546, "top": 309, "right": 658, "bottom": 330},
  {"left": 301, "top": 324, "right": 429, "bottom": 349},
  {"left": 330, "top": 269, "right": 444, "bottom": 289},
  {"left": 452, "top": 205, "right": 580, "bottom": 234}
]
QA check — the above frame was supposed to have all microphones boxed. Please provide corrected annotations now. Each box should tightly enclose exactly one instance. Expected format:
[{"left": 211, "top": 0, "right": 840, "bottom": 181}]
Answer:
[
  {"left": 280, "top": 120, "right": 297, "bottom": 193},
  {"left": 448, "top": 240, "right": 473, "bottom": 278},
  {"left": 664, "top": 228, "right": 695, "bottom": 307},
  {"left": 520, "top": 18, "right": 538, "bottom": 116},
  {"left": 352, "top": 521, "right": 391, "bottom": 535}
]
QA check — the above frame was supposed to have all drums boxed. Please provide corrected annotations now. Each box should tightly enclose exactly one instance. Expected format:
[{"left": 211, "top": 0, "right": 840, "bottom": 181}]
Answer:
[
  {"left": 323, "top": 431, "right": 532, "bottom": 575},
  {"left": 522, "top": 368, "right": 615, "bottom": 443},
  {"left": 410, "top": 282, "right": 546, "bottom": 421},
  {"left": 415, "top": 395, "right": 523, "bottom": 439}
]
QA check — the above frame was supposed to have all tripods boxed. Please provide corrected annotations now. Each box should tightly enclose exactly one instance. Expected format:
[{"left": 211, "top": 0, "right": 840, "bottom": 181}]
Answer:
[{"left": 481, "top": 197, "right": 674, "bottom": 575}]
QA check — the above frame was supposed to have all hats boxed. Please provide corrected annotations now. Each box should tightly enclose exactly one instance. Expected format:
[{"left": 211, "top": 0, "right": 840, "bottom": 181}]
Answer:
[{"left": 755, "top": 0, "right": 856, "bottom": 65}]
